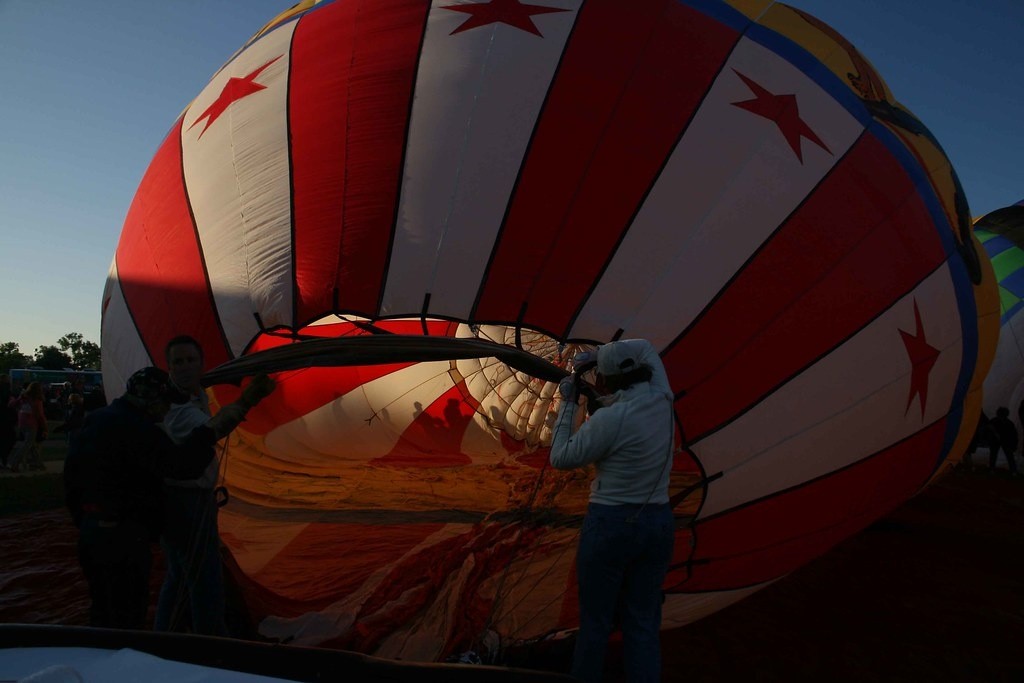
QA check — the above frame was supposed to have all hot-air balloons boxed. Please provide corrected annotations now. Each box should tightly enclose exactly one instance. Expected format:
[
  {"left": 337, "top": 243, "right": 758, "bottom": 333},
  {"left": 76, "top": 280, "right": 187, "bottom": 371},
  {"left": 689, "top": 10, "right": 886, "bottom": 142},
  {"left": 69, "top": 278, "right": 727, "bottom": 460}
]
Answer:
[
  {"left": 971, "top": 226, "right": 1024, "bottom": 474},
  {"left": 99, "top": 2, "right": 1000, "bottom": 659}
]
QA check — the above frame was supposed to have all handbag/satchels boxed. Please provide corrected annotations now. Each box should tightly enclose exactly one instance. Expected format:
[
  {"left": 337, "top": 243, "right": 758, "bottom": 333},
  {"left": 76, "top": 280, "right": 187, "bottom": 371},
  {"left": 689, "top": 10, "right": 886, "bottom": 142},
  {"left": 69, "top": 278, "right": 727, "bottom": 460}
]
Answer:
[{"left": 36, "top": 426, "right": 45, "bottom": 442}]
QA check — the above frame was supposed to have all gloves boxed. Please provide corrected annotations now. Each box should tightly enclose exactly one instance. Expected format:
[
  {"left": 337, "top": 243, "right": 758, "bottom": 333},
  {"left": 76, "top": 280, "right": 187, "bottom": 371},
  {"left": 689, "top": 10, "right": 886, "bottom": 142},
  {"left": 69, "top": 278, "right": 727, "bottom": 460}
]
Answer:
[
  {"left": 242, "top": 369, "right": 275, "bottom": 407},
  {"left": 571, "top": 352, "right": 597, "bottom": 373},
  {"left": 559, "top": 375, "right": 580, "bottom": 405}
]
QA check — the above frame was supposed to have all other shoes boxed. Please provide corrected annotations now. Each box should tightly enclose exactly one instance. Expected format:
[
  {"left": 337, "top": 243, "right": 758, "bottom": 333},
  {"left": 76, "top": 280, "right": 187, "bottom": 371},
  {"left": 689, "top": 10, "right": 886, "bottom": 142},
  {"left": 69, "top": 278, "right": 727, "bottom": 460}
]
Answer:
[{"left": 6, "top": 464, "right": 18, "bottom": 473}]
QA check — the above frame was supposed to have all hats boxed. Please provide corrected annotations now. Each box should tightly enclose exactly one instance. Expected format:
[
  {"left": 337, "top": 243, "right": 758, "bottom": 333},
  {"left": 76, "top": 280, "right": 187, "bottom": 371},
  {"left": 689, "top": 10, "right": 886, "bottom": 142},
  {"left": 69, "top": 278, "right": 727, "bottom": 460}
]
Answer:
[
  {"left": 596, "top": 342, "right": 639, "bottom": 377},
  {"left": 127, "top": 367, "right": 175, "bottom": 409}
]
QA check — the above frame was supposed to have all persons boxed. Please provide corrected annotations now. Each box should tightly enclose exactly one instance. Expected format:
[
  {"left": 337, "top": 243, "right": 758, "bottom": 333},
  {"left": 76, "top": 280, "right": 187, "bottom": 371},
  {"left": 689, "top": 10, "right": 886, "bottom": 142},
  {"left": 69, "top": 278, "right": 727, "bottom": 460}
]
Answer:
[
  {"left": 987, "top": 407, "right": 1018, "bottom": 478},
  {"left": 0, "top": 374, "right": 71, "bottom": 473},
  {"left": 64, "top": 334, "right": 275, "bottom": 635},
  {"left": 548, "top": 339, "right": 675, "bottom": 683}
]
